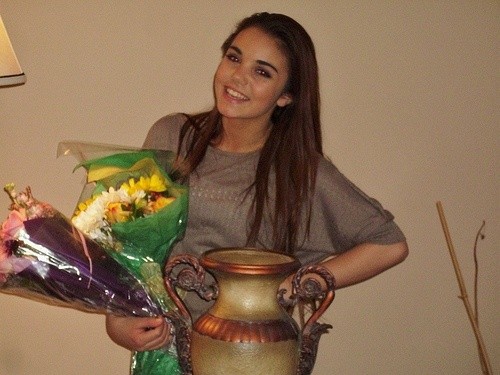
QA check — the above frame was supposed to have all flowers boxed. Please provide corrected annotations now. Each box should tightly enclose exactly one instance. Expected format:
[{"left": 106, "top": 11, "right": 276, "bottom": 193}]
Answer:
[
  {"left": 1, "top": 182, "right": 58, "bottom": 290},
  {"left": 71, "top": 171, "right": 176, "bottom": 252}
]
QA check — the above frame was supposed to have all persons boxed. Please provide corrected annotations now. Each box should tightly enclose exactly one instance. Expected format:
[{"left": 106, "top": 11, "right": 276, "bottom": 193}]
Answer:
[{"left": 103, "top": 12, "right": 411, "bottom": 375}]
